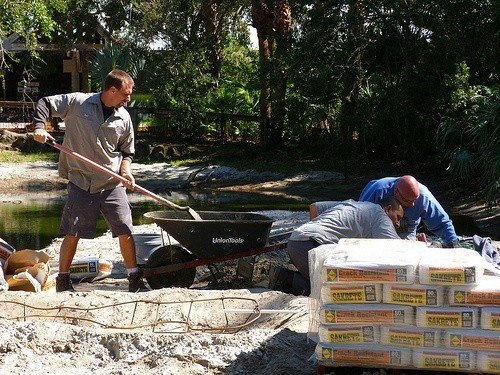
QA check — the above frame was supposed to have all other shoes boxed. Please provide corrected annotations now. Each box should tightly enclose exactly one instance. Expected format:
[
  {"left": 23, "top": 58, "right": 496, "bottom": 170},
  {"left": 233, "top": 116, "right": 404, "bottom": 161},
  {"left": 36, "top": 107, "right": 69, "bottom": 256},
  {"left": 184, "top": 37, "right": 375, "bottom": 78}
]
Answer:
[
  {"left": 128, "top": 271, "right": 153, "bottom": 293},
  {"left": 268, "top": 265, "right": 284, "bottom": 290},
  {"left": 56, "top": 273, "right": 76, "bottom": 293}
]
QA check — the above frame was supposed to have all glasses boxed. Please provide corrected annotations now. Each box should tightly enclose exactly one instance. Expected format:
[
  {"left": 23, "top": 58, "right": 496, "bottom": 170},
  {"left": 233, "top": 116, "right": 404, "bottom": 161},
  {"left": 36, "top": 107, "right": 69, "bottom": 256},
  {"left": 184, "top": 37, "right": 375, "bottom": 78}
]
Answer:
[{"left": 397, "top": 189, "right": 418, "bottom": 204}]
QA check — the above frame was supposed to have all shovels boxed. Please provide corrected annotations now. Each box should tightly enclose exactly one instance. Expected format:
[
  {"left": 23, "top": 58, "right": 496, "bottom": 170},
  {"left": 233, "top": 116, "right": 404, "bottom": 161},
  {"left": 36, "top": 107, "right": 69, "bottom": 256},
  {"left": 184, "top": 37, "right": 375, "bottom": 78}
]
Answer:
[{"left": 43, "top": 137, "right": 204, "bottom": 221}]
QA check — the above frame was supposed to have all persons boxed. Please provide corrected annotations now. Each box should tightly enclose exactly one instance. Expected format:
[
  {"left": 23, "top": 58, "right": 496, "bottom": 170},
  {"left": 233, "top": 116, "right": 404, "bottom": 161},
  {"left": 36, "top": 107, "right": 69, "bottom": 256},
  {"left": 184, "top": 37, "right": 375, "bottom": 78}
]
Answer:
[
  {"left": 267, "top": 197, "right": 409, "bottom": 297},
  {"left": 32, "top": 69, "right": 150, "bottom": 294},
  {"left": 359, "top": 176, "right": 461, "bottom": 250}
]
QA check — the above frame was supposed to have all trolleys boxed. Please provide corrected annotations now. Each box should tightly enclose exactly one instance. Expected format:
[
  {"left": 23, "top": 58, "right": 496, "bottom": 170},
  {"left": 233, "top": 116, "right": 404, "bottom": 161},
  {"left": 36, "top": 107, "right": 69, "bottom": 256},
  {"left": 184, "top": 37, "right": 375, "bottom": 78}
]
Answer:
[{"left": 139, "top": 211, "right": 300, "bottom": 289}]
